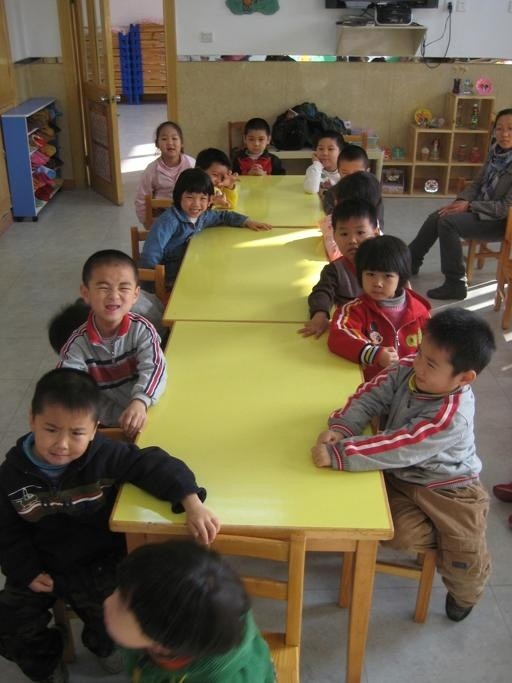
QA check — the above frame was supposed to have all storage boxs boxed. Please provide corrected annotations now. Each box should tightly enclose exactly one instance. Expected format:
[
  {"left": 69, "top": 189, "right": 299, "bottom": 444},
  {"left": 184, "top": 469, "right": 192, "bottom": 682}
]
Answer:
[
  {"left": 381, "top": 168, "right": 405, "bottom": 193},
  {"left": 351, "top": 132, "right": 379, "bottom": 148}
]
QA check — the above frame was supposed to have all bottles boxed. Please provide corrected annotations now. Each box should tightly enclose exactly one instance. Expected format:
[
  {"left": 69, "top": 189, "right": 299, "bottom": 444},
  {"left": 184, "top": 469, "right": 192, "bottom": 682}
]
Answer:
[
  {"left": 464, "top": 78, "right": 471, "bottom": 92},
  {"left": 453, "top": 144, "right": 481, "bottom": 162},
  {"left": 430, "top": 137, "right": 440, "bottom": 160},
  {"left": 470, "top": 102, "right": 480, "bottom": 130},
  {"left": 455, "top": 105, "right": 463, "bottom": 128}
]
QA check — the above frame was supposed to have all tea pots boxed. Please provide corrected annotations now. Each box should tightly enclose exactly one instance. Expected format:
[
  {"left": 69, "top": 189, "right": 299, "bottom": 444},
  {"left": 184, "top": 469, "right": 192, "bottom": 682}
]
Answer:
[{"left": 389, "top": 141, "right": 406, "bottom": 158}]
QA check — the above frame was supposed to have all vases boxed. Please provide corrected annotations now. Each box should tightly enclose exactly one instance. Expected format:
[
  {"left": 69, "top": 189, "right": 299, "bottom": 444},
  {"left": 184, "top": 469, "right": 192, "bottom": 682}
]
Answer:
[{"left": 451, "top": 77, "right": 462, "bottom": 93}]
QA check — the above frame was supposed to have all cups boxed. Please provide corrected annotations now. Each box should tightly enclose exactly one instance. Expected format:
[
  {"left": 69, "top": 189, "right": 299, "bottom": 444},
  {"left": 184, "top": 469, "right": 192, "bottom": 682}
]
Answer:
[
  {"left": 420, "top": 147, "right": 429, "bottom": 160},
  {"left": 452, "top": 77, "right": 462, "bottom": 94}
]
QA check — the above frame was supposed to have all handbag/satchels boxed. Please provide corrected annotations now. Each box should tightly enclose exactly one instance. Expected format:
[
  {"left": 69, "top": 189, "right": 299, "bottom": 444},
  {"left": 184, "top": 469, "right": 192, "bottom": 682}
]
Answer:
[{"left": 272, "top": 113, "right": 307, "bottom": 150}]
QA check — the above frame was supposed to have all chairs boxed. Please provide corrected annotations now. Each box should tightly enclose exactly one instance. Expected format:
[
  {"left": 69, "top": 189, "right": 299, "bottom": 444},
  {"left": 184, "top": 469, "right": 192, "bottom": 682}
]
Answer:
[
  {"left": 454, "top": 173, "right": 505, "bottom": 282},
  {"left": 224, "top": 121, "right": 249, "bottom": 157},
  {"left": 336, "top": 410, "right": 440, "bottom": 625},
  {"left": 52, "top": 426, "right": 143, "bottom": 664},
  {"left": 123, "top": 527, "right": 306, "bottom": 680},
  {"left": 337, "top": 130, "right": 369, "bottom": 153},
  {"left": 492, "top": 203, "right": 512, "bottom": 331}
]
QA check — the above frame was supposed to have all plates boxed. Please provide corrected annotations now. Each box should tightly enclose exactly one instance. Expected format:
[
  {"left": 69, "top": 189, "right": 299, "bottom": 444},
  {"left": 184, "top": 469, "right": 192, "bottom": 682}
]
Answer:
[
  {"left": 475, "top": 78, "right": 492, "bottom": 95},
  {"left": 414, "top": 108, "right": 432, "bottom": 126},
  {"left": 424, "top": 179, "right": 438, "bottom": 193}
]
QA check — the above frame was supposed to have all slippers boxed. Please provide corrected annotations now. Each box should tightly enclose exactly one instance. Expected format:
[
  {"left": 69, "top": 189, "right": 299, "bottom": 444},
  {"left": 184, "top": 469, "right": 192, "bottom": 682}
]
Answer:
[{"left": 29, "top": 108, "right": 63, "bottom": 200}]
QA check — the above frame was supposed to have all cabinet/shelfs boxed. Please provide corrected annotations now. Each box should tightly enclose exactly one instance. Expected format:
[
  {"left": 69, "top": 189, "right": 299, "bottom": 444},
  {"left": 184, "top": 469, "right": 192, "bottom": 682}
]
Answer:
[
  {"left": 381, "top": 93, "right": 496, "bottom": 197},
  {"left": 2, "top": 95, "right": 63, "bottom": 222}
]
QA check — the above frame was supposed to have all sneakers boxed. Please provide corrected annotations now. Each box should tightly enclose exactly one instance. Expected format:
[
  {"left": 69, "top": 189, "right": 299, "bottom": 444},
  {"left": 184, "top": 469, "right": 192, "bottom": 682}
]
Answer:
[
  {"left": 445, "top": 593, "right": 473, "bottom": 621},
  {"left": 493, "top": 482, "right": 512, "bottom": 504},
  {"left": 427, "top": 283, "right": 468, "bottom": 300},
  {"left": 47, "top": 625, "right": 69, "bottom": 683},
  {"left": 99, "top": 651, "right": 124, "bottom": 673}
]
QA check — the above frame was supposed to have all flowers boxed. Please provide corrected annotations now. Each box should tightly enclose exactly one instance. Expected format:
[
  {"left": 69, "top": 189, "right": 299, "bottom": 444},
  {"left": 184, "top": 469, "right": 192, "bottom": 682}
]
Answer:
[{"left": 452, "top": 63, "right": 467, "bottom": 79}]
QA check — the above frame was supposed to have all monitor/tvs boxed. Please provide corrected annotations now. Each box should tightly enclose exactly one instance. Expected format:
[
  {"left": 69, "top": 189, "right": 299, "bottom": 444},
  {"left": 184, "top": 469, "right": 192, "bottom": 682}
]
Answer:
[{"left": 319, "top": 0, "right": 445, "bottom": 26}]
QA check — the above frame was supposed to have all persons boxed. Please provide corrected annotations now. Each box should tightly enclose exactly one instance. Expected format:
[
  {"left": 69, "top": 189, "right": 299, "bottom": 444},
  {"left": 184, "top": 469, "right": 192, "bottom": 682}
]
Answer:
[
  {"left": 297, "top": 197, "right": 380, "bottom": 342},
  {"left": 323, "top": 145, "right": 371, "bottom": 216},
  {"left": 403, "top": 108, "right": 512, "bottom": 301},
  {"left": 303, "top": 130, "right": 344, "bottom": 197},
  {"left": 231, "top": 118, "right": 286, "bottom": 176},
  {"left": 48, "top": 287, "right": 170, "bottom": 357},
  {"left": 55, "top": 249, "right": 167, "bottom": 439},
  {"left": 321, "top": 169, "right": 381, "bottom": 262},
  {"left": 101, "top": 539, "right": 278, "bottom": 683},
  {"left": 135, "top": 121, "right": 196, "bottom": 230},
  {"left": 195, "top": 147, "right": 240, "bottom": 211},
  {"left": 0, "top": 368, "right": 222, "bottom": 683},
  {"left": 307, "top": 305, "right": 497, "bottom": 622},
  {"left": 135, "top": 168, "right": 272, "bottom": 301},
  {"left": 326, "top": 234, "right": 432, "bottom": 383}
]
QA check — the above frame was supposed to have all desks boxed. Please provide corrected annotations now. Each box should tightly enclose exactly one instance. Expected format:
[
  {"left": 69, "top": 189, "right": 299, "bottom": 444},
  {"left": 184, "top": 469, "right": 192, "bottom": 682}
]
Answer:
[
  {"left": 230, "top": 175, "right": 327, "bottom": 228},
  {"left": 266, "top": 143, "right": 384, "bottom": 183},
  {"left": 109, "top": 320, "right": 395, "bottom": 680},
  {"left": 161, "top": 227, "right": 339, "bottom": 331}
]
